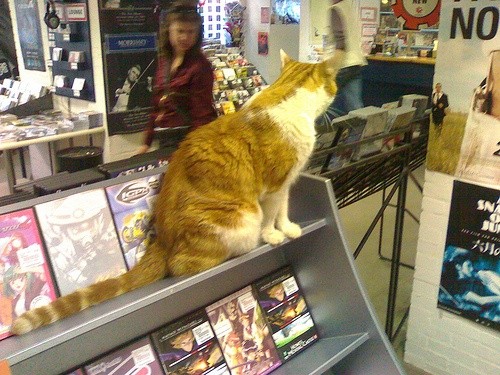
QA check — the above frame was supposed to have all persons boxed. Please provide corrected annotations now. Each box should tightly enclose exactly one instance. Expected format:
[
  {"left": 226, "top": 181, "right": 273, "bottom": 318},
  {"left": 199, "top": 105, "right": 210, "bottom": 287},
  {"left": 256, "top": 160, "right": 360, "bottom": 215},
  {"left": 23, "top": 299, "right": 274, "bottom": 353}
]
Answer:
[
  {"left": 322, "top": 0, "right": 367, "bottom": 124},
  {"left": 136, "top": 0, "right": 218, "bottom": 153},
  {"left": 431, "top": 83, "right": 449, "bottom": 128},
  {"left": 111, "top": 63, "right": 153, "bottom": 113}
]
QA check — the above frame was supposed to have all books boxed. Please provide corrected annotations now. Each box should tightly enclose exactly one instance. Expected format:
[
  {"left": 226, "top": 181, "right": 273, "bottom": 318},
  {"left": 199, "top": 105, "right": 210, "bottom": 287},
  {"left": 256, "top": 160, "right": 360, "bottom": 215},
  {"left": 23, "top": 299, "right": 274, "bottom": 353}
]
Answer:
[
  {"left": 202, "top": 42, "right": 267, "bottom": 115},
  {"left": 0, "top": 147, "right": 322, "bottom": 374},
  {"left": 306, "top": 93, "right": 430, "bottom": 175},
  {"left": 0, "top": 78, "right": 104, "bottom": 143}
]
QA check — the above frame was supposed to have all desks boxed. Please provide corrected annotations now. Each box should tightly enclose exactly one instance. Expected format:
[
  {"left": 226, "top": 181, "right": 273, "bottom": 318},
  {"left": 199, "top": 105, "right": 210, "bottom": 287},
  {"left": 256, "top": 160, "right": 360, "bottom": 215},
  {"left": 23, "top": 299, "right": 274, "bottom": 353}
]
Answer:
[
  {"left": 366, "top": 54, "right": 436, "bottom": 106},
  {"left": 0, "top": 123, "right": 105, "bottom": 186}
]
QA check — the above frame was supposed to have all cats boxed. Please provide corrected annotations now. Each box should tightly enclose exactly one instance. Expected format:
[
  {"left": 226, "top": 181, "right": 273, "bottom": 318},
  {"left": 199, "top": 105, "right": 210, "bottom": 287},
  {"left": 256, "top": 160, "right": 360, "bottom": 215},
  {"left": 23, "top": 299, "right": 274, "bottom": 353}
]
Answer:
[{"left": 8, "top": 49, "right": 347, "bottom": 337}]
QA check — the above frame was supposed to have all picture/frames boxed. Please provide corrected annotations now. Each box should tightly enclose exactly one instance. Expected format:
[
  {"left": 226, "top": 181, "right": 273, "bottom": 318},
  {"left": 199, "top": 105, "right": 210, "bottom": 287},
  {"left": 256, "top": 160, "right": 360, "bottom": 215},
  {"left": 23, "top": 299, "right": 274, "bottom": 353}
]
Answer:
[{"left": 360, "top": 7, "right": 377, "bottom": 21}]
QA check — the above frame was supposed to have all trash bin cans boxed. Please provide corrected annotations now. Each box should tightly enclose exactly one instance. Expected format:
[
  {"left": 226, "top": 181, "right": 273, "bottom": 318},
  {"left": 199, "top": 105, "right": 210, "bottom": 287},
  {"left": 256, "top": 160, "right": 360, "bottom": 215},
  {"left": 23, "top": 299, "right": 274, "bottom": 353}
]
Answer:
[{"left": 56, "top": 146, "right": 103, "bottom": 172}]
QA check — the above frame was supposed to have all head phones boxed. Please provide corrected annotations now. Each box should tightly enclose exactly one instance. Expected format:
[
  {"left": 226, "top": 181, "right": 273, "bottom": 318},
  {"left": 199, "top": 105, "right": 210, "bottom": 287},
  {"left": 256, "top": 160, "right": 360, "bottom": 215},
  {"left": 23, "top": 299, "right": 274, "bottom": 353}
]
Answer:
[{"left": 44, "top": 0, "right": 60, "bottom": 29}]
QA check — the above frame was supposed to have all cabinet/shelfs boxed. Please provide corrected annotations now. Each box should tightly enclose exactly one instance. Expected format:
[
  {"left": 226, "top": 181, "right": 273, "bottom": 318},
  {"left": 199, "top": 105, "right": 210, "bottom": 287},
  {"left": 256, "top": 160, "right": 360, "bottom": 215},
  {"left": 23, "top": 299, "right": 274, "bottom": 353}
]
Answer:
[{"left": 0, "top": 166, "right": 408, "bottom": 375}]
